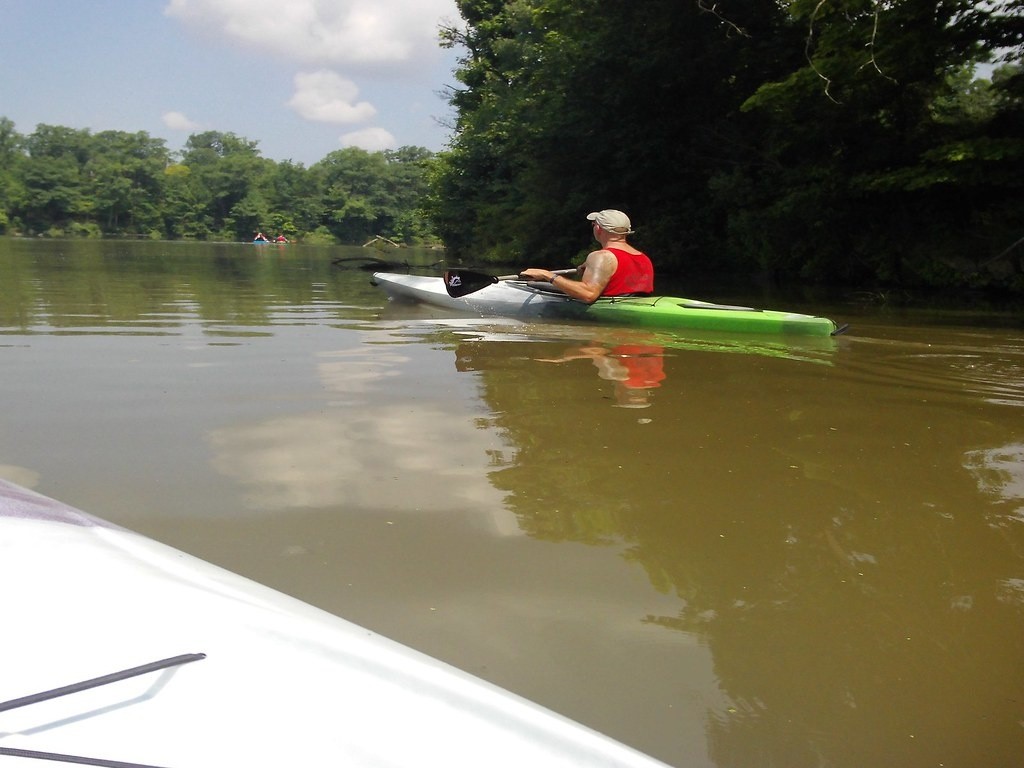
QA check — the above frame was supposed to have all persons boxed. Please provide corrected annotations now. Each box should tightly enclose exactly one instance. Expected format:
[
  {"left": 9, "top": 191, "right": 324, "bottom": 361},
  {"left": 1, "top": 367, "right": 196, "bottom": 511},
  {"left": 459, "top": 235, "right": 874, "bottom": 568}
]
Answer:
[
  {"left": 534, "top": 338, "right": 665, "bottom": 408},
  {"left": 275, "top": 233, "right": 288, "bottom": 243},
  {"left": 253, "top": 232, "right": 267, "bottom": 241},
  {"left": 520, "top": 209, "right": 653, "bottom": 303}
]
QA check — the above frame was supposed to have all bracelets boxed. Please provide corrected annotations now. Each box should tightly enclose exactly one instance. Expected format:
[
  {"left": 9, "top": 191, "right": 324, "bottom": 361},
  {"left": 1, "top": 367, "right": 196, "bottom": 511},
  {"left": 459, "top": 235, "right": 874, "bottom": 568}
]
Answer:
[{"left": 550, "top": 274, "right": 559, "bottom": 284}]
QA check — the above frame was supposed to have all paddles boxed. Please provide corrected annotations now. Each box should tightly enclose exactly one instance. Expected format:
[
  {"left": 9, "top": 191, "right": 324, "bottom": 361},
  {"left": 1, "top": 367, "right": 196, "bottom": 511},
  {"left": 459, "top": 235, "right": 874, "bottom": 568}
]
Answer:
[{"left": 442, "top": 268, "right": 579, "bottom": 299}]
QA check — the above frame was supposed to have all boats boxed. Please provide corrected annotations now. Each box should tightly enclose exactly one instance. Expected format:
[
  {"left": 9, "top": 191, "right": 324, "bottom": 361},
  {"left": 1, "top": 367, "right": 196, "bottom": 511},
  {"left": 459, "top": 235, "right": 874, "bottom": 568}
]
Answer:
[
  {"left": 277, "top": 241, "right": 289, "bottom": 244},
  {"left": 253, "top": 241, "right": 268, "bottom": 244},
  {"left": 0, "top": 481, "right": 670, "bottom": 768},
  {"left": 370, "top": 272, "right": 849, "bottom": 337}
]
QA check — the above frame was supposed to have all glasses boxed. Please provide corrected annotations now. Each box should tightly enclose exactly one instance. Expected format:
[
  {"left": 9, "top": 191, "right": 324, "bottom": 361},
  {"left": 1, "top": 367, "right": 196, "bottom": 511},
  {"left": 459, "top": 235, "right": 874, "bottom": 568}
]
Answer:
[{"left": 591, "top": 221, "right": 597, "bottom": 226}]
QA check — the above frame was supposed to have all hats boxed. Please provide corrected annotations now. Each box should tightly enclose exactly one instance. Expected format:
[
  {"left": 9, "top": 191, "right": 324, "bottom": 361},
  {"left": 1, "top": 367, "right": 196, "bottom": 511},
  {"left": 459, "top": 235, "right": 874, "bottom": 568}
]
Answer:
[{"left": 586, "top": 209, "right": 635, "bottom": 235}]
